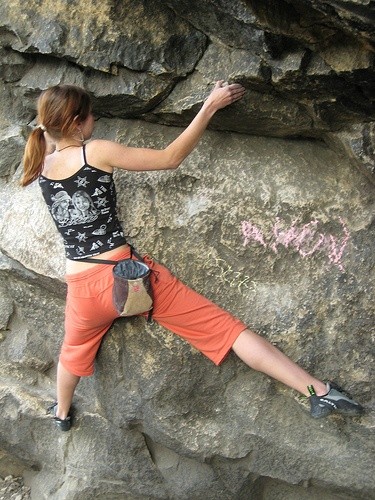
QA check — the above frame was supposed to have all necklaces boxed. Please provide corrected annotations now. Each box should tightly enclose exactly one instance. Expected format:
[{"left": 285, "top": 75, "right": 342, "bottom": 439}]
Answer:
[{"left": 53, "top": 144, "right": 82, "bottom": 152}]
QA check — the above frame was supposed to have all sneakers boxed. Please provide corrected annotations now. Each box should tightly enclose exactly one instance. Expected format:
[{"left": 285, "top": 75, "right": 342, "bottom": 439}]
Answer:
[
  {"left": 44, "top": 401, "right": 73, "bottom": 431},
  {"left": 306, "top": 378, "right": 363, "bottom": 421}
]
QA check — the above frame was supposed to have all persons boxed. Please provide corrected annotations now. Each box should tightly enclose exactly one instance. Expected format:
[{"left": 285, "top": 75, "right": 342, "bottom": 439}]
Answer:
[{"left": 18, "top": 78, "right": 364, "bottom": 431}]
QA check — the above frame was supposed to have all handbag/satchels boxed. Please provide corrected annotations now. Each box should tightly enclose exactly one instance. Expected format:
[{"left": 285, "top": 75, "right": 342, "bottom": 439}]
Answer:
[{"left": 111, "top": 259, "right": 159, "bottom": 318}]
